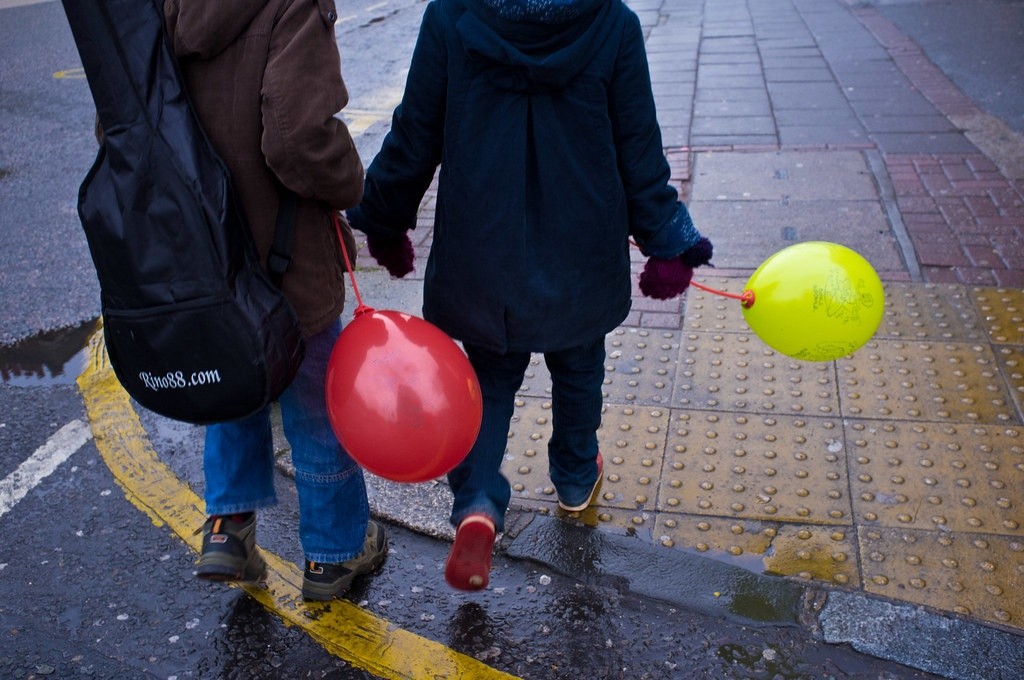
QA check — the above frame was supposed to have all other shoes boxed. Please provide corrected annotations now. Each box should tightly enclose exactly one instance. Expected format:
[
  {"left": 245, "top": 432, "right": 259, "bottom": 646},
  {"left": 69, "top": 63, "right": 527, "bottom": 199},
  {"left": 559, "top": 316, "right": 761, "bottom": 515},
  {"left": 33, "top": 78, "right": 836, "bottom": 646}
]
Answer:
[
  {"left": 559, "top": 450, "right": 603, "bottom": 511},
  {"left": 445, "top": 512, "right": 495, "bottom": 590}
]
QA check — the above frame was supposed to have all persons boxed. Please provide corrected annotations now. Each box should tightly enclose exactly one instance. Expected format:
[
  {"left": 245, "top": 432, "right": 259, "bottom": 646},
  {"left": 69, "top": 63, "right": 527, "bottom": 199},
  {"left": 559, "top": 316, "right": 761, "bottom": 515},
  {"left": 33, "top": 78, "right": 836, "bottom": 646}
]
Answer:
[
  {"left": 367, "top": 0, "right": 714, "bottom": 590},
  {"left": 157, "top": 0, "right": 389, "bottom": 602}
]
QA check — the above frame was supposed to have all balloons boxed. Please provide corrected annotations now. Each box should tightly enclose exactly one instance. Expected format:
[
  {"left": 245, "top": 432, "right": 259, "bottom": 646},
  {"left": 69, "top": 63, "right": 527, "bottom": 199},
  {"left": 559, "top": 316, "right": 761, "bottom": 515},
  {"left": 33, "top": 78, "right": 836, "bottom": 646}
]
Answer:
[
  {"left": 328, "top": 310, "right": 483, "bottom": 483},
  {"left": 740, "top": 241, "right": 886, "bottom": 363}
]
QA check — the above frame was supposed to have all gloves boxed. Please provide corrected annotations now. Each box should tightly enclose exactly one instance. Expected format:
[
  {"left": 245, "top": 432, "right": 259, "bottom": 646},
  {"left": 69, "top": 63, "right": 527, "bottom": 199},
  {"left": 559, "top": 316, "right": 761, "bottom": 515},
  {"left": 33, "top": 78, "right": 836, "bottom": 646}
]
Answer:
[
  {"left": 367, "top": 231, "right": 401, "bottom": 254},
  {"left": 679, "top": 237, "right": 713, "bottom": 268}
]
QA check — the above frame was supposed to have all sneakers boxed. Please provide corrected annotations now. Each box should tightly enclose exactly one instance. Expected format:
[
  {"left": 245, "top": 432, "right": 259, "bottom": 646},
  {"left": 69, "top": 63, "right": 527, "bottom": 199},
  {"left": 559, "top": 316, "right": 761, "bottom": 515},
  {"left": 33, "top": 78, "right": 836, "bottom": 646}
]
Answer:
[
  {"left": 193, "top": 512, "right": 268, "bottom": 581},
  {"left": 301, "top": 511, "right": 386, "bottom": 601}
]
